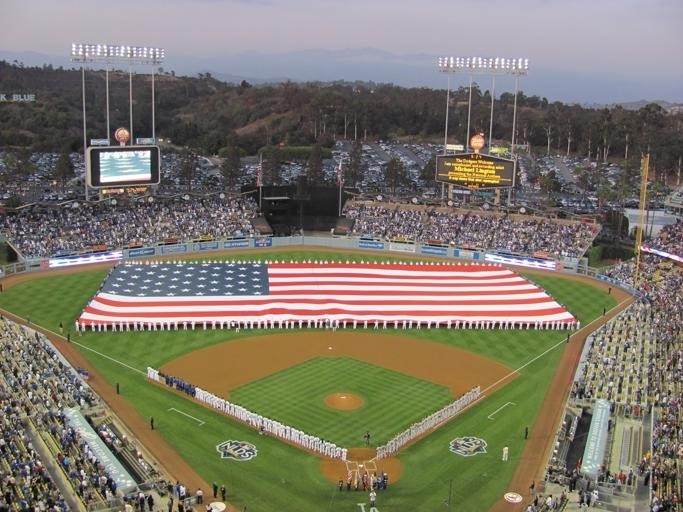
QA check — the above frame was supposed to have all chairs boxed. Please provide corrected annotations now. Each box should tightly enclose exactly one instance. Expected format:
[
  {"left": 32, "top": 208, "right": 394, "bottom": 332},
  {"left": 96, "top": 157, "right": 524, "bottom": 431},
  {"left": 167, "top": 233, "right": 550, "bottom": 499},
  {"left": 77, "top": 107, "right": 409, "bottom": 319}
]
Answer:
[
  {"left": 528, "top": 224, "right": 683, "bottom": 512},
  {"left": 0, "top": 312, "right": 180, "bottom": 512}
]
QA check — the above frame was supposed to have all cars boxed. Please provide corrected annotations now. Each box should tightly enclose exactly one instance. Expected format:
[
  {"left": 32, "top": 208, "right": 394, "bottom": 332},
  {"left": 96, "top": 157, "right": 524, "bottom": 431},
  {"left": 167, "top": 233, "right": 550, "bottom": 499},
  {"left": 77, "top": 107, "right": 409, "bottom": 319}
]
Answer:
[
  {"left": 327, "top": 135, "right": 453, "bottom": 186},
  {"left": 513, "top": 150, "right": 670, "bottom": 215},
  {"left": 0, "top": 151, "right": 84, "bottom": 201},
  {"left": 159, "top": 151, "right": 327, "bottom": 186}
]
